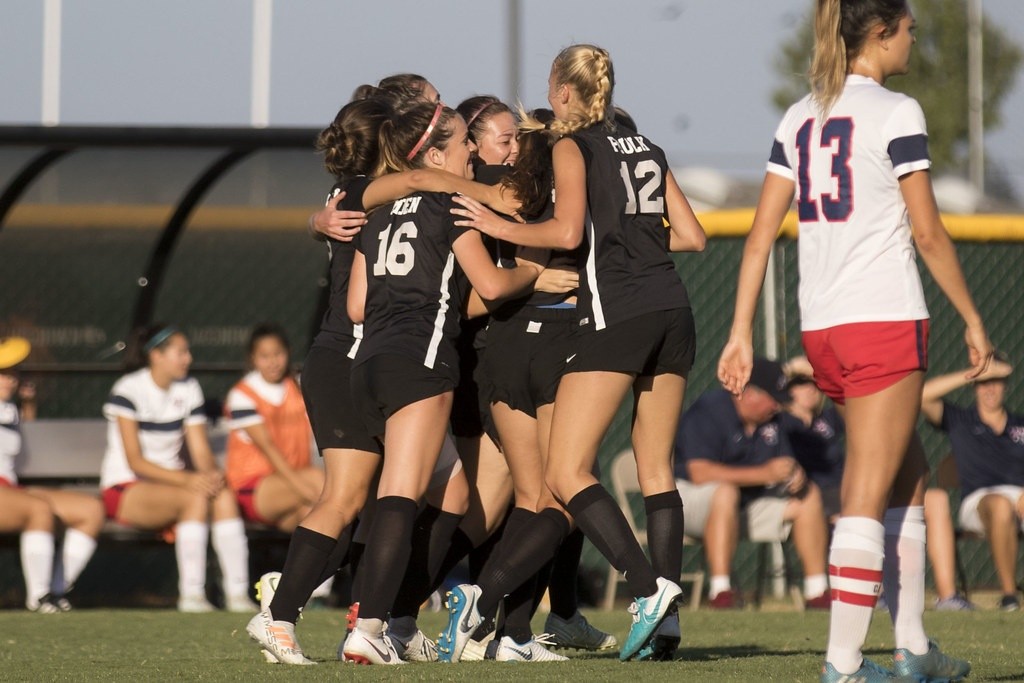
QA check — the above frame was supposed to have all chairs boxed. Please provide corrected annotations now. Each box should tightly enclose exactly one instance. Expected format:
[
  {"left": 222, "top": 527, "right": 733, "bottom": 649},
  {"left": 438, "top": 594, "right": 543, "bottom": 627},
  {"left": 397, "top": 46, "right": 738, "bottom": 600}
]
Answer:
[
  {"left": 602, "top": 448, "right": 743, "bottom": 614},
  {"left": 936, "top": 451, "right": 1021, "bottom": 606}
]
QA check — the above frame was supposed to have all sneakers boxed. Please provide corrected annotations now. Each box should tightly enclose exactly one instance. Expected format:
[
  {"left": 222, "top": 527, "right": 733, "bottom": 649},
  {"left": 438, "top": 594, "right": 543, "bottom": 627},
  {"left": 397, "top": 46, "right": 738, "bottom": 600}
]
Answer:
[
  {"left": 620, "top": 576, "right": 683, "bottom": 661},
  {"left": 459, "top": 630, "right": 496, "bottom": 661},
  {"left": 246, "top": 611, "right": 317, "bottom": 665},
  {"left": 636, "top": 609, "right": 681, "bottom": 661},
  {"left": 342, "top": 627, "right": 409, "bottom": 665},
  {"left": 893, "top": 638, "right": 971, "bottom": 683},
  {"left": 255, "top": 571, "right": 304, "bottom": 663},
  {"left": 384, "top": 628, "right": 438, "bottom": 662},
  {"left": 435, "top": 584, "right": 485, "bottom": 664},
  {"left": 495, "top": 633, "right": 571, "bottom": 661},
  {"left": 821, "top": 656, "right": 895, "bottom": 683},
  {"left": 544, "top": 610, "right": 616, "bottom": 652}
]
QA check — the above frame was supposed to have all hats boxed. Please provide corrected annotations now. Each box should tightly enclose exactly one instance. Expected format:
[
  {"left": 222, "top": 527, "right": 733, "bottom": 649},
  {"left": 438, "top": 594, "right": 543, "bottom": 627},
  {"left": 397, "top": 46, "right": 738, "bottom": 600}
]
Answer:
[
  {"left": 750, "top": 359, "right": 793, "bottom": 402},
  {"left": 0, "top": 336, "right": 30, "bottom": 368}
]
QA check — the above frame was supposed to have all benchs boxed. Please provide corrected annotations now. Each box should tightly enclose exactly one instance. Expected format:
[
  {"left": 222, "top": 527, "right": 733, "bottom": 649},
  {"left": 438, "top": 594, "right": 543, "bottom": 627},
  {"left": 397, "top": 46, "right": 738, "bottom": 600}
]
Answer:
[{"left": 0, "top": 415, "right": 291, "bottom": 610}]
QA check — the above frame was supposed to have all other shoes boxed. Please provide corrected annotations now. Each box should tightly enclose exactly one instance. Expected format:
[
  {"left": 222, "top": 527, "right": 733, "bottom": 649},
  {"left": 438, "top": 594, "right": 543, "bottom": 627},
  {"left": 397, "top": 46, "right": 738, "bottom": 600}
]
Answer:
[
  {"left": 935, "top": 597, "right": 978, "bottom": 612},
  {"left": 56, "top": 584, "right": 76, "bottom": 611},
  {"left": 708, "top": 591, "right": 736, "bottom": 610},
  {"left": 806, "top": 591, "right": 830, "bottom": 610},
  {"left": 24, "top": 593, "right": 62, "bottom": 612},
  {"left": 999, "top": 595, "right": 1019, "bottom": 612}
]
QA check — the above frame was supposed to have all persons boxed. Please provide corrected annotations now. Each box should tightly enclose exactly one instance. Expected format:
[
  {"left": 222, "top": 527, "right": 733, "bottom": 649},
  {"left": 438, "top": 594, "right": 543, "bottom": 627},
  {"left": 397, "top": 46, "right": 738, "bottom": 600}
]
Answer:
[
  {"left": 98, "top": 328, "right": 263, "bottom": 614},
  {"left": 451, "top": 43, "right": 708, "bottom": 661},
  {"left": 339, "top": 103, "right": 552, "bottom": 665},
  {"left": 484, "top": 130, "right": 571, "bottom": 665},
  {"left": 718, "top": 0, "right": 992, "bottom": 683},
  {"left": 244, "top": 73, "right": 639, "bottom": 673},
  {"left": 223, "top": 325, "right": 357, "bottom": 615},
  {"left": 673, "top": 358, "right": 849, "bottom": 608},
  {"left": 920, "top": 350, "right": 1024, "bottom": 611},
  {"left": 449, "top": 91, "right": 616, "bottom": 653},
  {"left": 0, "top": 336, "right": 105, "bottom": 615}
]
